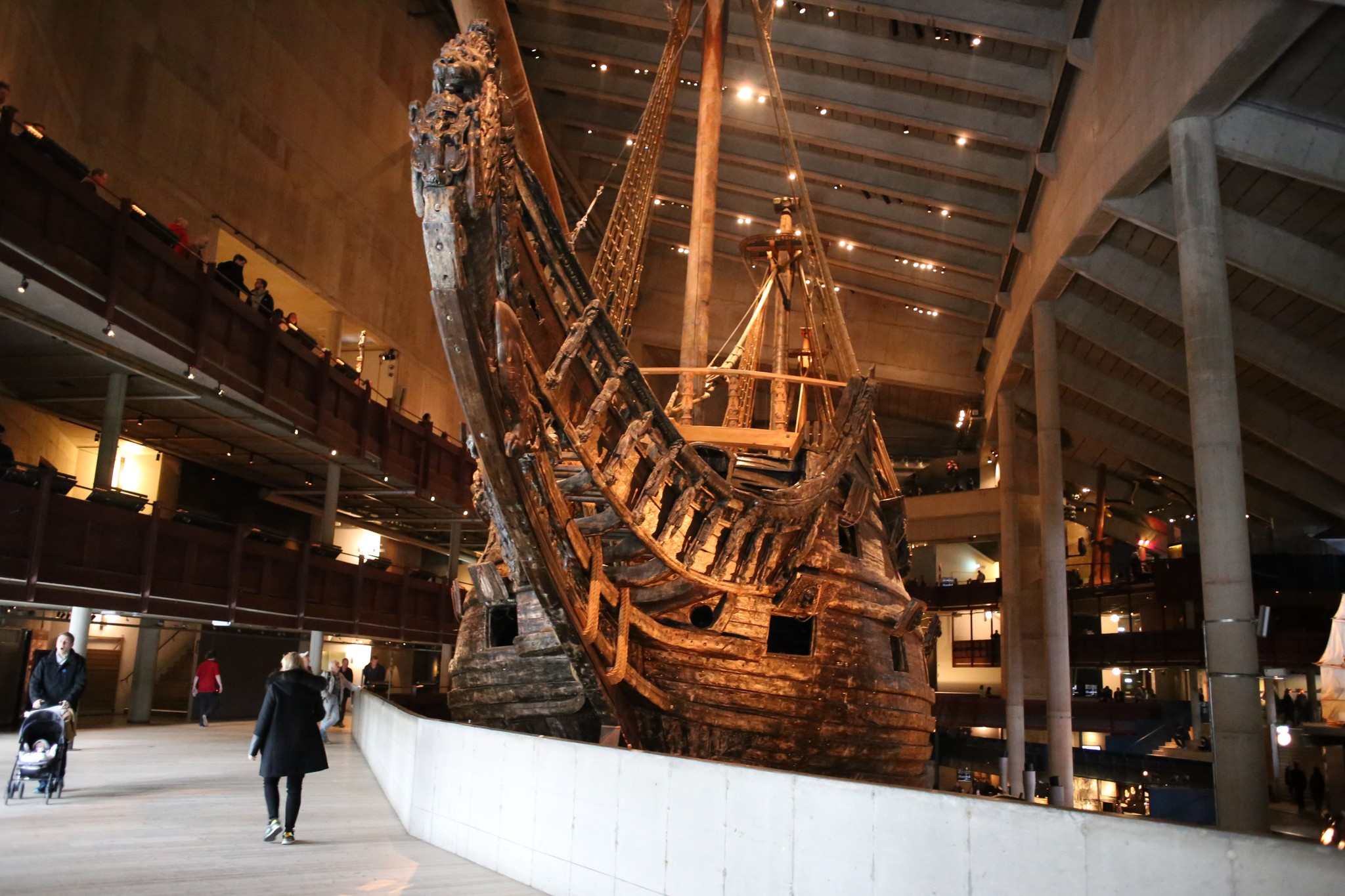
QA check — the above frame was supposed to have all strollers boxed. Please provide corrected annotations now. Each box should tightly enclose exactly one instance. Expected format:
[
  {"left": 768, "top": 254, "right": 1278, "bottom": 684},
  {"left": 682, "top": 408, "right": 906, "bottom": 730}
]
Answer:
[{"left": 4, "top": 700, "right": 77, "bottom": 805}]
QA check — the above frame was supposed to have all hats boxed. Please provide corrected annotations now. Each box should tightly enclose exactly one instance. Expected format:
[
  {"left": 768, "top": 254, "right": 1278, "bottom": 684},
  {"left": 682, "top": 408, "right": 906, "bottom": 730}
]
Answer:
[
  {"left": 33, "top": 739, "right": 50, "bottom": 749},
  {"left": 299, "top": 651, "right": 308, "bottom": 658}
]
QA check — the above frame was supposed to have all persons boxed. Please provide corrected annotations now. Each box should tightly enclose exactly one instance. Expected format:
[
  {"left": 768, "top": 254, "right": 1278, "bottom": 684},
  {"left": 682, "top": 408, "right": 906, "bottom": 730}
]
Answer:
[
  {"left": 87, "top": 168, "right": 109, "bottom": 197},
  {"left": 991, "top": 630, "right": 1000, "bottom": 640},
  {"left": 905, "top": 571, "right": 985, "bottom": 589},
  {"left": 192, "top": 649, "right": 223, "bottom": 727},
  {"left": 319, "top": 660, "right": 366, "bottom": 744},
  {"left": 904, "top": 482, "right": 973, "bottom": 497},
  {"left": 215, "top": 254, "right": 297, "bottom": 326},
  {"left": 0, "top": 424, "right": 16, "bottom": 479},
  {"left": 28, "top": 632, "right": 87, "bottom": 793},
  {"left": 248, "top": 652, "right": 329, "bottom": 844},
  {"left": 299, "top": 651, "right": 313, "bottom": 674},
  {"left": 980, "top": 685, "right": 992, "bottom": 699},
  {"left": 951, "top": 778, "right": 991, "bottom": 796},
  {"left": 1071, "top": 686, "right": 1325, "bottom": 812},
  {"left": 364, "top": 656, "right": 386, "bottom": 686},
  {"left": 422, "top": 413, "right": 431, "bottom": 422},
  {"left": 0, "top": 81, "right": 10, "bottom": 104},
  {"left": 14, "top": 122, "right": 45, "bottom": 136},
  {"left": 167, "top": 218, "right": 210, "bottom": 272},
  {"left": 332, "top": 658, "right": 353, "bottom": 728}
]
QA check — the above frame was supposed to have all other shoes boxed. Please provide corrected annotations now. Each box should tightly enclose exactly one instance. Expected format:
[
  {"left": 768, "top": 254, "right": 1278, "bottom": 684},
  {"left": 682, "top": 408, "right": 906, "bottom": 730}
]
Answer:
[
  {"left": 339, "top": 722, "right": 345, "bottom": 728},
  {"left": 34, "top": 782, "right": 53, "bottom": 793},
  {"left": 333, "top": 722, "right": 338, "bottom": 726},
  {"left": 323, "top": 740, "right": 331, "bottom": 744},
  {"left": 202, "top": 715, "right": 208, "bottom": 727}
]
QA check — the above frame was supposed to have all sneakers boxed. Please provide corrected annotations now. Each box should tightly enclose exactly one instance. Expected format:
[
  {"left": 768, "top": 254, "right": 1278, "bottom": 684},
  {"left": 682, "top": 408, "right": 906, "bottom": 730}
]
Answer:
[
  {"left": 264, "top": 820, "right": 282, "bottom": 841},
  {"left": 281, "top": 831, "right": 295, "bottom": 844}
]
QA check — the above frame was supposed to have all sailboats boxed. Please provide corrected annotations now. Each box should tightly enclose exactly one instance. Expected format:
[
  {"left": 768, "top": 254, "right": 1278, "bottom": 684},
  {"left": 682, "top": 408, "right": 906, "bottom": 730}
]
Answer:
[{"left": 409, "top": 0, "right": 938, "bottom": 790}]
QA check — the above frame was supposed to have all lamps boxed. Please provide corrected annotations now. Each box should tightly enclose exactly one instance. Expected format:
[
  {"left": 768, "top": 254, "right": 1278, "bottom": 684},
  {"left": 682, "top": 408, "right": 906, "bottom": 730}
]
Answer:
[
  {"left": 249, "top": 454, "right": 468, "bottom": 541},
  {"left": 17, "top": 275, "right": 29, "bottom": 294},
  {"left": 156, "top": 453, "right": 161, "bottom": 462},
  {"left": 173, "top": 427, "right": 181, "bottom": 438},
  {"left": 382, "top": 348, "right": 396, "bottom": 360},
  {"left": 1253, "top": 603, "right": 1272, "bottom": 639},
  {"left": 183, "top": 365, "right": 195, "bottom": 380},
  {"left": 1062, "top": 463, "right": 1203, "bottom": 524},
  {"left": 94, "top": 433, "right": 101, "bottom": 443},
  {"left": 227, "top": 446, "right": 235, "bottom": 457},
  {"left": 137, "top": 415, "right": 146, "bottom": 427},
  {"left": 292, "top": 428, "right": 298, "bottom": 433},
  {"left": 103, "top": 321, "right": 114, "bottom": 336},
  {"left": 792, "top": 0, "right": 981, "bottom": 317},
  {"left": 885, "top": 447, "right": 998, "bottom": 471},
  {"left": 215, "top": 378, "right": 224, "bottom": 396},
  {"left": 6, "top": 606, "right": 96, "bottom": 622}
]
